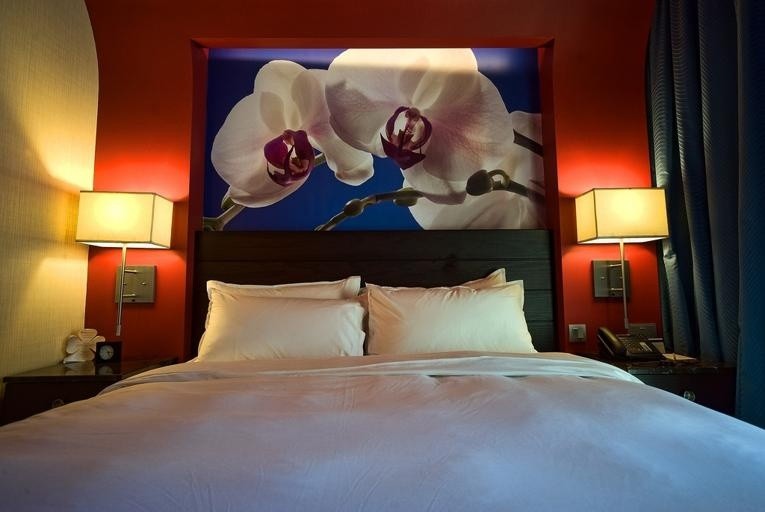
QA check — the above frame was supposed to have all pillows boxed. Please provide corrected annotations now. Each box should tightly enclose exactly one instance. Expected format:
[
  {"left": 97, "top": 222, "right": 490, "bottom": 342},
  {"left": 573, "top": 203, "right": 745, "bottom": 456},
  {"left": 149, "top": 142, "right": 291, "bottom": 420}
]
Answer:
[
  {"left": 354, "top": 263, "right": 508, "bottom": 361},
  {"left": 204, "top": 273, "right": 364, "bottom": 306},
  {"left": 362, "top": 279, "right": 539, "bottom": 363},
  {"left": 188, "top": 285, "right": 373, "bottom": 361}
]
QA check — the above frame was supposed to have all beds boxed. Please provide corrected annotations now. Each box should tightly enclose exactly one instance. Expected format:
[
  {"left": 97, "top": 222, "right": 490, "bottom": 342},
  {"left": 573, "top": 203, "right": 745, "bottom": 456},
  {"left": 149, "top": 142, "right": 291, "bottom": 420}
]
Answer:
[{"left": 0, "top": 224, "right": 765, "bottom": 509}]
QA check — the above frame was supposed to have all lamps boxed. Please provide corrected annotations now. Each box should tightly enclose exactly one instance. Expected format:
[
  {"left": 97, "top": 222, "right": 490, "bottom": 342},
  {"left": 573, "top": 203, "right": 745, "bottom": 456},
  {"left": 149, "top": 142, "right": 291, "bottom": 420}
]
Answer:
[
  {"left": 72, "top": 186, "right": 177, "bottom": 339},
  {"left": 573, "top": 184, "right": 677, "bottom": 334}
]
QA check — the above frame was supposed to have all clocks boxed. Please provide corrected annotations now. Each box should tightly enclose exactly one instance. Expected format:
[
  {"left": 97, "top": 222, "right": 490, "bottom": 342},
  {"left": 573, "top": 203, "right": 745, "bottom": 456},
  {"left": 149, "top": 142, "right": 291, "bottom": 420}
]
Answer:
[{"left": 92, "top": 339, "right": 123, "bottom": 364}]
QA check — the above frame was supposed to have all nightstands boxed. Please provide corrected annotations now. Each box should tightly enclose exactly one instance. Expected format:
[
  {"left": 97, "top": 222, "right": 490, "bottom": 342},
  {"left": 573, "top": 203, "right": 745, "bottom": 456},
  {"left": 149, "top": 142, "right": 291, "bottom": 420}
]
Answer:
[
  {"left": 1, "top": 355, "right": 174, "bottom": 434},
  {"left": 570, "top": 340, "right": 739, "bottom": 423}
]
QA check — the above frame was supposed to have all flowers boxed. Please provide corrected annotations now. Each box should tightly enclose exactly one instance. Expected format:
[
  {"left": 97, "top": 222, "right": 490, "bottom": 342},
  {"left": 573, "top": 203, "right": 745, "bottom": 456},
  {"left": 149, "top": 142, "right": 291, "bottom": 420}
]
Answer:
[{"left": 200, "top": 46, "right": 550, "bottom": 236}]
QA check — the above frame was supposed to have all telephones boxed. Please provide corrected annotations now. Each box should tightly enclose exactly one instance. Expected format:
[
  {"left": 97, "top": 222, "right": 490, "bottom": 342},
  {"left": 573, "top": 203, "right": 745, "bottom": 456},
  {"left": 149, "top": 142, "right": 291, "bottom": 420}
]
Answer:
[{"left": 596, "top": 326, "right": 663, "bottom": 361}]
